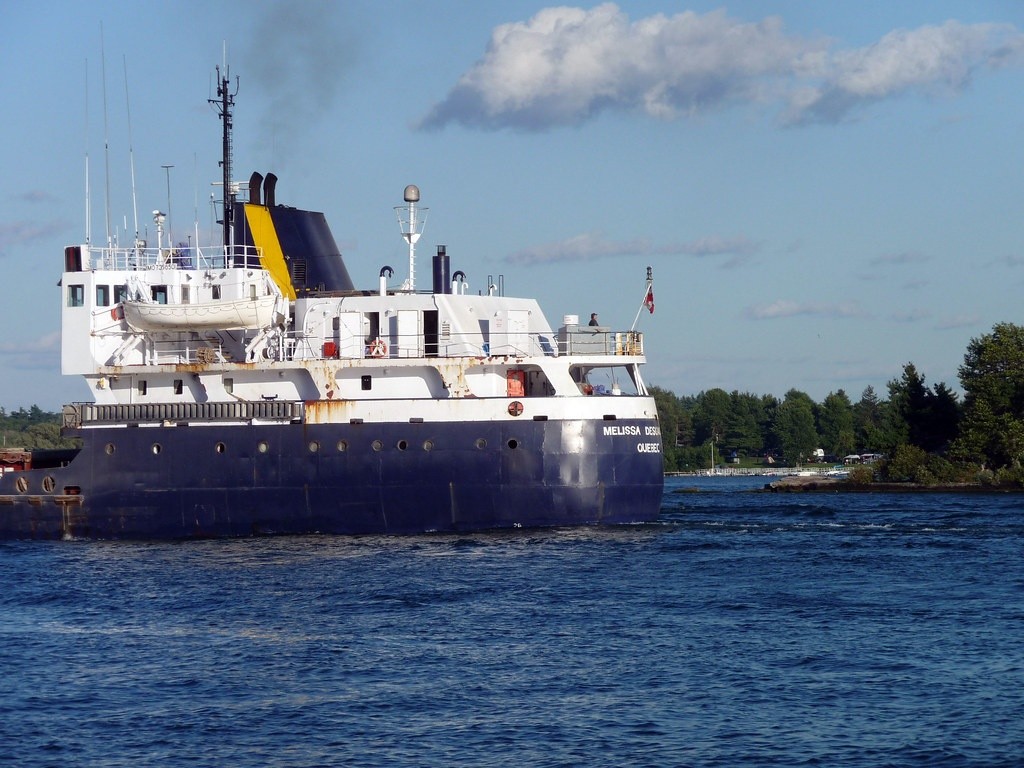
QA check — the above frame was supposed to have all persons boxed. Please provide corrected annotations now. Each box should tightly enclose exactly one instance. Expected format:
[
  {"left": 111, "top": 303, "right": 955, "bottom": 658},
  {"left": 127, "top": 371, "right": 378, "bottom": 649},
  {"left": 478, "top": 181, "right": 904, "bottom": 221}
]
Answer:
[{"left": 588, "top": 313, "right": 599, "bottom": 326}]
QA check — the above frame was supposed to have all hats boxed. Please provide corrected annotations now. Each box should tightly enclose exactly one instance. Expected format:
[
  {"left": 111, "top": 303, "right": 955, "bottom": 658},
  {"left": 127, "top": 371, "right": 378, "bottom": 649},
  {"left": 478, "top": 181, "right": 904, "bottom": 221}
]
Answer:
[{"left": 591, "top": 313, "right": 597, "bottom": 315}]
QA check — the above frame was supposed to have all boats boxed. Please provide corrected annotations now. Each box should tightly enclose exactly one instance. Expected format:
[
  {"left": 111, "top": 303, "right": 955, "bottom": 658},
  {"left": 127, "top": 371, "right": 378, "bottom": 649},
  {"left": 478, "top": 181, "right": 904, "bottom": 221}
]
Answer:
[{"left": 0, "top": 38, "right": 668, "bottom": 546}]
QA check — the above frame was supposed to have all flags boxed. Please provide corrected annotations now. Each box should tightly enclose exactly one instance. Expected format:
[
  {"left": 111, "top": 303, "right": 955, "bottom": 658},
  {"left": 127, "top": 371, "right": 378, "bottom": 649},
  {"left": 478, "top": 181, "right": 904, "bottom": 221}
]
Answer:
[{"left": 643, "top": 286, "right": 654, "bottom": 314}]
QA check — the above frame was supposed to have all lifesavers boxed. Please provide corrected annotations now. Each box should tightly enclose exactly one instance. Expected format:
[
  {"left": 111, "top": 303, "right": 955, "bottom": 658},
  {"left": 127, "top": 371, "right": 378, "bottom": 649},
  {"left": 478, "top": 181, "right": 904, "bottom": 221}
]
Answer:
[
  {"left": 370, "top": 340, "right": 386, "bottom": 357},
  {"left": 111, "top": 303, "right": 125, "bottom": 321}
]
